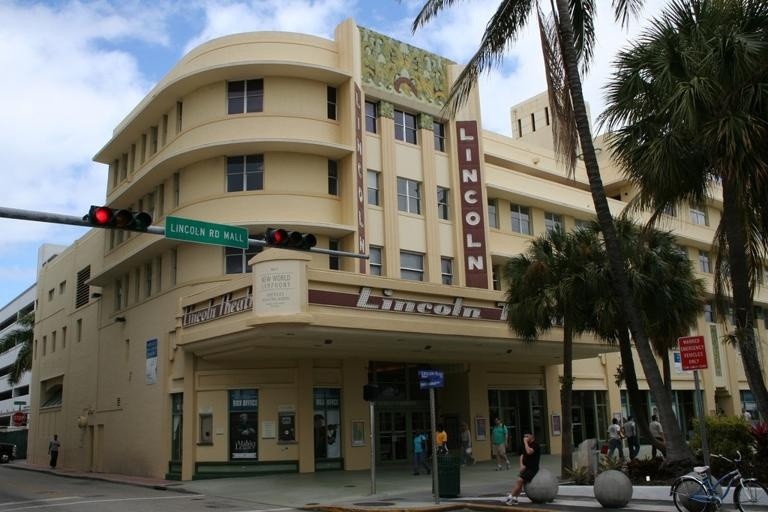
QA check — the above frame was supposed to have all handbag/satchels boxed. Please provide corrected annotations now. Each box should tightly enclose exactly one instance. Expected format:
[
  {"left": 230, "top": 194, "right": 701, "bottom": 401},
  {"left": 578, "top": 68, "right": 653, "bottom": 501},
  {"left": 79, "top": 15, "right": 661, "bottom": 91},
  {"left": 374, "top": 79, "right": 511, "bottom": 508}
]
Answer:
[
  {"left": 601, "top": 445, "right": 608, "bottom": 454},
  {"left": 421, "top": 440, "right": 426, "bottom": 449}
]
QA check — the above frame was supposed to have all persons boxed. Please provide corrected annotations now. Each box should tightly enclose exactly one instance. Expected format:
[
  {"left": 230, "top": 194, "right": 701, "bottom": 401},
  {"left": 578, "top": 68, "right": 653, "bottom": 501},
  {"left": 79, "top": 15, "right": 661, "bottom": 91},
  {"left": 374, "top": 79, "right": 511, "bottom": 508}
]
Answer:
[
  {"left": 740, "top": 407, "right": 752, "bottom": 426},
  {"left": 622, "top": 415, "right": 640, "bottom": 460},
  {"left": 605, "top": 417, "right": 626, "bottom": 466},
  {"left": 411, "top": 426, "right": 431, "bottom": 476},
  {"left": 435, "top": 424, "right": 448, "bottom": 453},
  {"left": 314, "top": 413, "right": 336, "bottom": 461},
  {"left": 47, "top": 432, "right": 62, "bottom": 469},
  {"left": 502, "top": 430, "right": 542, "bottom": 506},
  {"left": 237, "top": 414, "right": 255, "bottom": 440},
  {"left": 649, "top": 414, "right": 667, "bottom": 459},
  {"left": 717, "top": 409, "right": 728, "bottom": 418},
  {"left": 455, "top": 423, "right": 476, "bottom": 468},
  {"left": 715, "top": 407, "right": 722, "bottom": 415},
  {"left": 491, "top": 416, "right": 511, "bottom": 472}
]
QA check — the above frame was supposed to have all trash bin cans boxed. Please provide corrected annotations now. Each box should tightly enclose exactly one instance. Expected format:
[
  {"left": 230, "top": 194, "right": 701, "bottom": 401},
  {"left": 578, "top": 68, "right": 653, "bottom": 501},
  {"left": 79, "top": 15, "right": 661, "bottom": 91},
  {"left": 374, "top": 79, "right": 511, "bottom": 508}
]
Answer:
[{"left": 438, "top": 454, "right": 460, "bottom": 498}]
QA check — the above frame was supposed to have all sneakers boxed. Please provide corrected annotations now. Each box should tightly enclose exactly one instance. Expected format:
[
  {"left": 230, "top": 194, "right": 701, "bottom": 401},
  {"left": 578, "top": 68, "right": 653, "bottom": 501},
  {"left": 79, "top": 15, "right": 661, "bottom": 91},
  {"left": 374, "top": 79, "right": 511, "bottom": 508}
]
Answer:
[
  {"left": 496, "top": 467, "right": 503, "bottom": 471},
  {"left": 503, "top": 497, "right": 511, "bottom": 502},
  {"left": 471, "top": 458, "right": 475, "bottom": 464},
  {"left": 507, "top": 465, "right": 511, "bottom": 470},
  {"left": 465, "top": 462, "right": 471, "bottom": 466},
  {"left": 506, "top": 499, "right": 519, "bottom": 506}
]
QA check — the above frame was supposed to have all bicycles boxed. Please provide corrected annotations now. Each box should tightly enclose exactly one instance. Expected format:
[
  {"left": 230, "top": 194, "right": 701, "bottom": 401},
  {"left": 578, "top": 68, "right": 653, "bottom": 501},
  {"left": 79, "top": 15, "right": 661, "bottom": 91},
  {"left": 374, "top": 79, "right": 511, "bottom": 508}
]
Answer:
[{"left": 670, "top": 450, "right": 768, "bottom": 512}]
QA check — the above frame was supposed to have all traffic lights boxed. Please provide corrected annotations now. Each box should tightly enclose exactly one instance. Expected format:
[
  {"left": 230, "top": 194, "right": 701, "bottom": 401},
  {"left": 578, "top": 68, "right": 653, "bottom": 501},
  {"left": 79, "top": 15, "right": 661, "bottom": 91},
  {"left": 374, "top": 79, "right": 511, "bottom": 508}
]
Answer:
[
  {"left": 362, "top": 384, "right": 378, "bottom": 401},
  {"left": 265, "top": 226, "right": 318, "bottom": 251},
  {"left": 88, "top": 205, "right": 153, "bottom": 233}
]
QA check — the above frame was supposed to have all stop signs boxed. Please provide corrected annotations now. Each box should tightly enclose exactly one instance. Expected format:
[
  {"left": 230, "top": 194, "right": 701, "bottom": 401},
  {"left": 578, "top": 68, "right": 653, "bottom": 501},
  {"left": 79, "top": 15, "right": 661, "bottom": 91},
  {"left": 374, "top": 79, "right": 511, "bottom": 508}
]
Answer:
[{"left": 12, "top": 411, "right": 26, "bottom": 425}]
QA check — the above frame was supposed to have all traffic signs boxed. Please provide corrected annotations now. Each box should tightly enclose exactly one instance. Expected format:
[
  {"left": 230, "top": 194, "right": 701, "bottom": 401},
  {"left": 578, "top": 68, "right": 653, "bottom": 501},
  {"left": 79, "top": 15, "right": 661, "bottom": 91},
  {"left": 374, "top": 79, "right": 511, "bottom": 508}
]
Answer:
[{"left": 165, "top": 216, "right": 249, "bottom": 251}]
